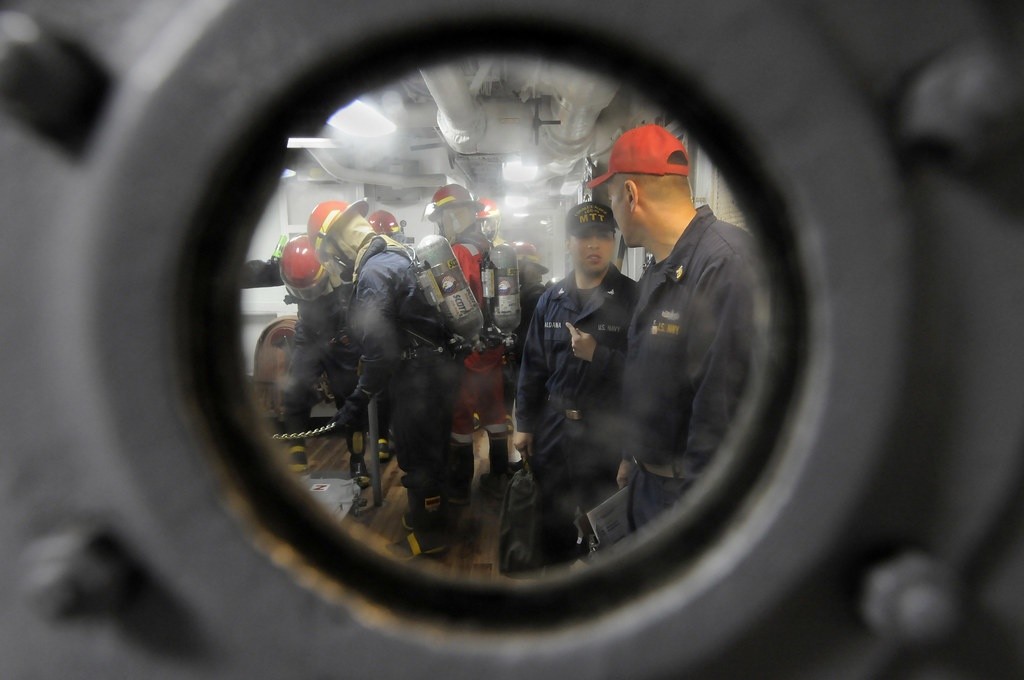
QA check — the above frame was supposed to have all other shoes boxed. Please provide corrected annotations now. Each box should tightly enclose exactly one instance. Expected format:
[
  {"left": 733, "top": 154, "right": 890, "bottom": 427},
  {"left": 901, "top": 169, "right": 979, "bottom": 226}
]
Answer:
[
  {"left": 473, "top": 412, "right": 481, "bottom": 429},
  {"left": 508, "top": 462, "right": 521, "bottom": 475},
  {"left": 385, "top": 532, "right": 447, "bottom": 562},
  {"left": 401, "top": 512, "right": 461, "bottom": 533},
  {"left": 378, "top": 438, "right": 390, "bottom": 462},
  {"left": 290, "top": 446, "right": 311, "bottom": 481},
  {"left": 401, "top": 474, "right": 409, "bottom": 488},
  {"left": 479, "top": 473, "right": 509, "bottom": 499},
  {"left": 506, "top": 415, "right": 514, "bottom": 433},
  {"left": 350, "top": 456, "right": 370, "bottom": 487}
]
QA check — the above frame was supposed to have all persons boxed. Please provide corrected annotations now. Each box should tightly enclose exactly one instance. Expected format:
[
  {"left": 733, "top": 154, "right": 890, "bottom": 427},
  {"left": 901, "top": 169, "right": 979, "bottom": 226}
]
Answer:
[
  {"left": 511, "top": 201, "right": 640, "bottom": 569},
  {"left": 586, "top": 124, "right": 769, "bottom": 532},
  {"left": 242, "top": 184, "right": 545, "bottom": 560}
]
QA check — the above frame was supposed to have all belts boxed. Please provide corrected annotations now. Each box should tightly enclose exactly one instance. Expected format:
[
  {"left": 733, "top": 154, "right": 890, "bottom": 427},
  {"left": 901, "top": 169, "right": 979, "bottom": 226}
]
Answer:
[
  {"left": 630, "top": 454, "right": 705, "bottom": 481},
  {"left": 549, "top": 400, "right": 614, "bottom": 420}
]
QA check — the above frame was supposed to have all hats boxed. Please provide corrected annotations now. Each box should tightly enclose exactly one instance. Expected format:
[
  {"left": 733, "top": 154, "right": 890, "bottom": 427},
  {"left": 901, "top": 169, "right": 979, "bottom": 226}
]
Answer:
[
  {"left": 587, "top": 125, "right": 689, "bottom": 188},
  {"left": 567, "top": 202, "right": 617, "bottom": 236}
]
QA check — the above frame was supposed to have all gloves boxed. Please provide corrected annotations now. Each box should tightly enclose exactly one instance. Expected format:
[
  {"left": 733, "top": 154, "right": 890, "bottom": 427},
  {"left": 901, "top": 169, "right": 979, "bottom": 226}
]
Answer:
[{"left": 326, "top": 367, "right": 382, "bottom": 428}]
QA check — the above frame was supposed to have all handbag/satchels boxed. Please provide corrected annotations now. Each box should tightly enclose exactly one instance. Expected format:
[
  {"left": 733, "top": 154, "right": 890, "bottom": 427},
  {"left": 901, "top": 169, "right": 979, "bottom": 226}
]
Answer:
[{"left": 498, "top": 451, "right": 545, "bottom": 579}]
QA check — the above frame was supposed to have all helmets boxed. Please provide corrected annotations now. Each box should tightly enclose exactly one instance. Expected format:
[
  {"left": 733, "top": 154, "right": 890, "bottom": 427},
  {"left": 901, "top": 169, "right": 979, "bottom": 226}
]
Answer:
[
  {"left": 427, "top": 184, "right": 485, "bottom": 221},
  {"left": 475, "top": 197, "right": 499, "bottom": 219},
  {"left": 279, "top": 233, "right": 327, "bottom": 289},
  {"left": 368, "top": 210, "right": 401, "bottom": 235},
  {"left": 307, "top": 199, "right": 368, "bottom": 262}
]
